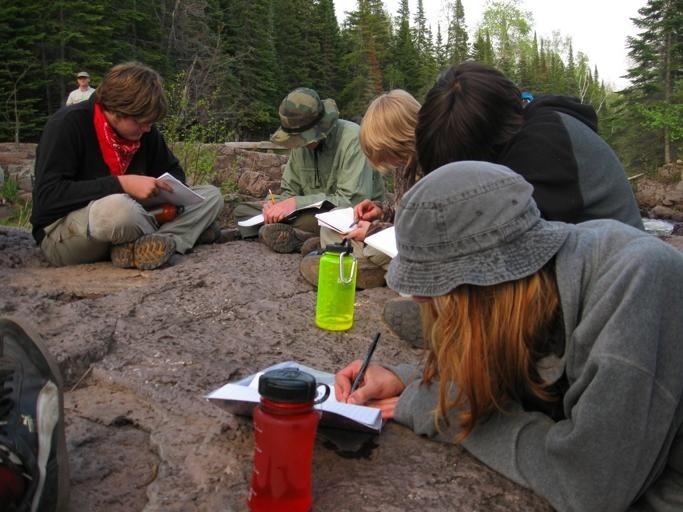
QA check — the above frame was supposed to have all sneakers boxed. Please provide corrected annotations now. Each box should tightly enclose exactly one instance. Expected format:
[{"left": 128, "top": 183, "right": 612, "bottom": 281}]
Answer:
[
  {"left": 301, "top": 236, "right": 320, "bottom": 257},
  {"left": 259, "top": 223, "right": 314, "bottom": 254},
  {"left": 381, "top": 297, "right": 431, "bottom": 350},
  {"left": 195, "top": 222, "right": 220, "bottom": 245},
  {"left": 110, "top": 233, "right": 177, "bottom": 270},
  {"left": 298, "top": 251, "right": 385, "bottom": 288}
]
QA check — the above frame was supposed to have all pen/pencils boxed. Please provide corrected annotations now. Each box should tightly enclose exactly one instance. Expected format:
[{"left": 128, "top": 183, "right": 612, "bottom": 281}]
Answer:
[
  {"left": 268, "top": 190, "right": 277, "bottom": 203},
  {"left": 345, "top": 332, "right": 379, "bottom": 403},
  {"left": 349, "top": 217, "right": 361, "bottom": 228}
]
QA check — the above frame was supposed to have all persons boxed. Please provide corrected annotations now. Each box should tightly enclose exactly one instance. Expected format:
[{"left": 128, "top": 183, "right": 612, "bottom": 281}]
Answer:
[
  {"left": 334, "top": 162, "right": 683, "bottom": 512},
  {"left": 233, "top": 87, "right": 378, "bottom": 248},
  {"left": 29, "top": 61, "right": 224, "bottom": 271},
  {"left": 66, "top": 72, "right": 95, "bottom": 105},
  {"left": 382, "top": 62, "right": 646, "bottom": 348},
  {"left": 300, "top": 88, "right": 422, "bottom": 289}
]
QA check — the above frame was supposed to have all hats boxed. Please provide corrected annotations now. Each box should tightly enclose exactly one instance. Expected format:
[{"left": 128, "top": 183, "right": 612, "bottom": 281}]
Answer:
[
  {"left": 77, "top": 71, "right": 90, "bottom": 78},
  {"left": 270, "top": 86, "right": 341, "bottom": 148},
  {"left": 385, "top": 160, "right": 569, "bottom": 298}
]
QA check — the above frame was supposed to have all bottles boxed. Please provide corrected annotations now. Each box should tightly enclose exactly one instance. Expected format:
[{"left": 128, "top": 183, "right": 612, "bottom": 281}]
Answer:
[
  {"left": 145, "top": 202, "right": 184, "bottom": 222},
  {"left": 313, "top": 237, "right": 359, "bottom": 332},
  {"left": 243, "top": 366, "right": 332, "bottom": 512}
]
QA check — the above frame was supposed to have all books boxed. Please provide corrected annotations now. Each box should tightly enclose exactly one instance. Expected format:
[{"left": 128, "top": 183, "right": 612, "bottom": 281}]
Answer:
[
  {"left": 238, "top": 200, "right": 337, "bottom": 227},
  {"left": 203, "top": 360, "right": 389, "bottom": 435},
  {"left": 315, "top": 207, "right": 359, "bottom": 235},
  {"left": 137, "top": 172, "right": 205, "bottom": 206},
  {"left": 363, "top": 226, "right": 397, "bottom": 259}
]
